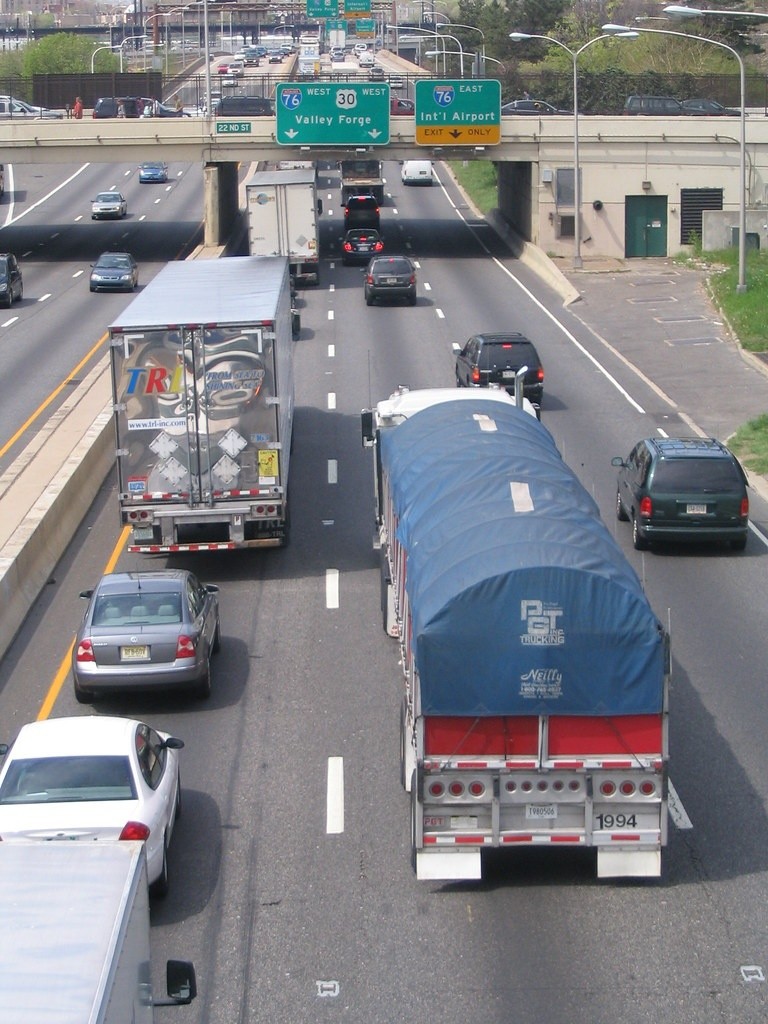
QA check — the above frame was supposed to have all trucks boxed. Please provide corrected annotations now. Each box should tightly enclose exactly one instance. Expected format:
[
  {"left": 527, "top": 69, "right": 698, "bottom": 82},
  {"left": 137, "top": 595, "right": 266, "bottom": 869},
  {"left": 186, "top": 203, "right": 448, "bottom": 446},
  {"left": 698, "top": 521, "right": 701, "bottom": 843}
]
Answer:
[
  {"left": 337, "top": 158, "right": 386, "bottom": 206},
  {"left": 359, "top": 364, "right": 696, "bottom": 893},
  {"left": 0, "top": 839, "right": 196, "bottom": 1023},
  {"left": 107, "top": 253, "right": 300, "bottom": 554},
  {"left": 246, "top": 170, "right": 322, "bottom": 280}
]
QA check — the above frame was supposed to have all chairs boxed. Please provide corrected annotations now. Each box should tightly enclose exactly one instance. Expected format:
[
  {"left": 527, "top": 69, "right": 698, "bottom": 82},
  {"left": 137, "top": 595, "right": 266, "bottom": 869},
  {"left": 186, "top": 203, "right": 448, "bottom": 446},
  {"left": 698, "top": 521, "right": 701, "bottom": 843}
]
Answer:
[{"left": 102, "top": 605, "right": 181, "bottom": 625}]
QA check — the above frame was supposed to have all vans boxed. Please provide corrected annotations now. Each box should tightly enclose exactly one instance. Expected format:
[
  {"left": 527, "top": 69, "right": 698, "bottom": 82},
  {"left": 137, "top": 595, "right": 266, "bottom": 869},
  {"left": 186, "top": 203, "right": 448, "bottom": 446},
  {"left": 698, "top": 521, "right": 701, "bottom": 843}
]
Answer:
[{"left": 399, "top": 160, "right": 435, "bottom": 186}]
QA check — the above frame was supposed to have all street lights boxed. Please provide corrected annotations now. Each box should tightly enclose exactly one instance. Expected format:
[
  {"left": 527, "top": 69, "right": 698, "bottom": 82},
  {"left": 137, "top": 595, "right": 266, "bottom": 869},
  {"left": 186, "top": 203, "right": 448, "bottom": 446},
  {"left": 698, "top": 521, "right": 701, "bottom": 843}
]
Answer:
[
  {"left": 386, "top": 25, "right": 447, "bottom": 79},
  {"left": 182, "top": 1, "right": 202, "bottom": 68},
  {"left": 219, "top": 2, "right": 236, "bottom": 51},
  {"left": 144, "top": 13, "right": 171, "bottom": 73},
  {"left": 198, "top": 0, "right": 216, "bottom": 58},
  {"left": 91, "top": 45, "right": 122, "bottom": 74},
  {"left": 400, "top": 34, "right": 464, "bottom": 80},
  {"left": 601, "top": 23, "right": 761, "bottom": 296},
  {"left": 166, "top": 6, "right": 190, "bottom": 74},
  {"left": 436, "top": 23, "right": 486, "bottom": 79},
  {"left": 509, "top": 30, "right": 639, "bottom": 271},
  {"left": 120, "top": 35, "right": 149, "bottom": 75}
]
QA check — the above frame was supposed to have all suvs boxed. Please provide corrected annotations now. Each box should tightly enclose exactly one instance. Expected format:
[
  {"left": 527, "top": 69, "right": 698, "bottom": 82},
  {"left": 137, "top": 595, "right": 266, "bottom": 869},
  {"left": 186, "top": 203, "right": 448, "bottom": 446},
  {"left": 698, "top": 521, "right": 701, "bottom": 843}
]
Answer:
[
  {"left": 622, "top": 93, "right": 710, "bottom": 118},
  {"left": 0, "top": 253, "right": 24, "bottom": 307},
  {"left": 340, "top": 194, "right": 381, "bottom": 230},
  {"left": 454, "top": 332, "right": 549, "bottom": 399},
  {"left": 0, "top": 96, "right": 64, "bottom": 119},
  {"left": 364, "top": 254, "right": 419, "bottom": 306},
  {"left": 610, "top": 435, "right": 751, "bottom": 547},
  {"left": 92, "top": 97, "right": 192, "bottom": 118},
  {"left": 212, "top": 95, "right": 274, "bottom": 117}
]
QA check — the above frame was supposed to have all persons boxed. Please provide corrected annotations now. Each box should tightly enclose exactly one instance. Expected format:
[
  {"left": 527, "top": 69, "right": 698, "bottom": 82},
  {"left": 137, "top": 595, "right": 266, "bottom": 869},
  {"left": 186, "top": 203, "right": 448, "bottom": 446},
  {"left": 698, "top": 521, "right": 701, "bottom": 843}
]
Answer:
[
  {"left": 174, "top": 95, "right": 184, "bottom": 116},
  {"left": 117, "top": 99, "right": 126, "bottom": 118},
  {"left": 136, "top": 95, "right": 160, "bottom": 117},
  {"left": 523, "top": 90, "right": 532, "bottom": 100},
  {"left": 73, "top": 97, "right": 82, "bottom": 119}
]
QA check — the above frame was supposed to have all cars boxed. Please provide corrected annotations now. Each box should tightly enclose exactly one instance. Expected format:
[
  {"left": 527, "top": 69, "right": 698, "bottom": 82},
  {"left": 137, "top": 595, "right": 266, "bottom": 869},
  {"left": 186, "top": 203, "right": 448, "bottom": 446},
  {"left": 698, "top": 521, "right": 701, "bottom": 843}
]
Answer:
[
  {"left": 0, "top": 164, "right": 5, "bottom": 194},
  {"left": 385, "top": 75, "right": 405, "bottom": 87},
  {"left": 75, "top": 567, "right": 220, "bottom": 703},
  {"left": 680, "top": 97, "right": 750, "bottom": 117},
  {"left": 501, "top": 98, "right": 574, "bottom": 117},
  {"left": 389, "top": 97, "right": 415, "bottom": 116},
  {"left": 0, "top": 716, "right": 185, "bottom": 895},
  {"left": 200, "top": 36, "right": 374, "bottom": 111},
  {"left": 339, "top": 228, "right": 385, "bottom": 263},
  {"left": 90, "top": 192, "right": 129, "bottom": 218},
  {"left": 368, "top": 67, "right": 385, "bottom": 81},
  {"left": 90, "top": 253, "right": 139, "bottom": 291},
  {"left": 278, "top": 160, "right": 318, "bottom": 177},
  {"left": 138, "top": 160, "right": 169, "bottom": 183}
]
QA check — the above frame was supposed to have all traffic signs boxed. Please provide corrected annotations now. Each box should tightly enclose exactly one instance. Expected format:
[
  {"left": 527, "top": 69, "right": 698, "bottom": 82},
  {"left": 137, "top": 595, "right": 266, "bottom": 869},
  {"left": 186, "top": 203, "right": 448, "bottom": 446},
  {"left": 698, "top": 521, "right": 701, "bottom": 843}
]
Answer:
[
  {"left": 412, "top": 79, "right": 507, "bottom": 152},
  {"left": 345, "top": 0, "right": 372, "bottom": 19},
  {"left": 306, "top": 0, "right": 338, "bottom": 18},
  {"left": 275, "top": 81, "right": 392, "bottom": 149}
]
